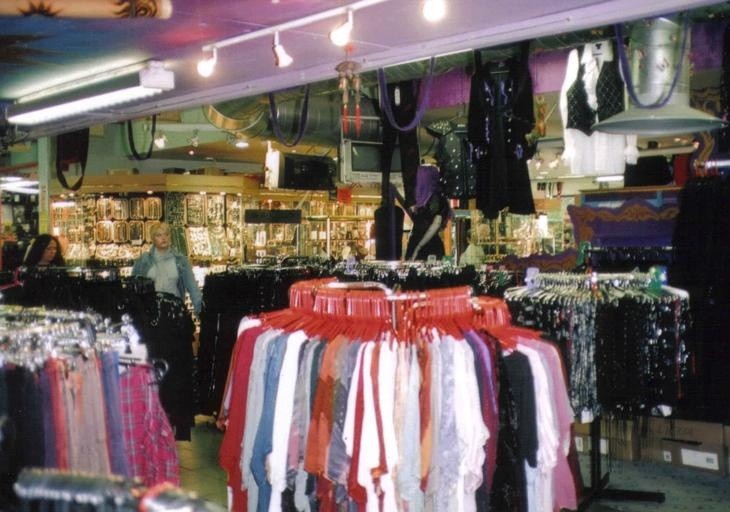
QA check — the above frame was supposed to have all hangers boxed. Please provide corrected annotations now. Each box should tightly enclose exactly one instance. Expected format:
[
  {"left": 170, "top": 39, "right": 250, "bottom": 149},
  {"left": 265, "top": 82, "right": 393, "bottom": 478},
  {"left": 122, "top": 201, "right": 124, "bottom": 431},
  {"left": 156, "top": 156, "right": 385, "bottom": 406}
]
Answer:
[
  {"left": 505, "top": 272, "right": 678, "bottom": 305},
  {"left": 259, "top": 277, "right": 541, "bottom": 351},
  {"left": 0, "top": 307, "right": 148, "bottom": 373}
]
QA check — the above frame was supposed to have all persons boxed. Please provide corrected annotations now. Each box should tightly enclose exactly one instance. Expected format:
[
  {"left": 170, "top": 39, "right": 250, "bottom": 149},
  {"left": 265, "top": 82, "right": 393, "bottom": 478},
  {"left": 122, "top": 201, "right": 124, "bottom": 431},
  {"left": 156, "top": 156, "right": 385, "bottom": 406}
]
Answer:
[
  {"left": 458, "top": 228, "right": 486, "bottom": 270},
  {"left": 131, "top": 223, "right": 204, "bottom": 358},
  {"left": 23, "top": 235, "right": 69, "bottom": 276},
  {"left": 403, "top": 161, "right": 453, "bottom": 265}
]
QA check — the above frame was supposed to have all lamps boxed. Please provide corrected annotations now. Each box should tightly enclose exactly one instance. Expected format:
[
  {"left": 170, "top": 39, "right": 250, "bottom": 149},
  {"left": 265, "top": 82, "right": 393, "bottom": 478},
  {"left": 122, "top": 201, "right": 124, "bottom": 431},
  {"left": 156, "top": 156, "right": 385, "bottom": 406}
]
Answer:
[
  {"left": 197, "top": 0, "right": 446, "bottom": 78},
  {"left": 5, "top": 59, "right": 175, "bottom": 126}
]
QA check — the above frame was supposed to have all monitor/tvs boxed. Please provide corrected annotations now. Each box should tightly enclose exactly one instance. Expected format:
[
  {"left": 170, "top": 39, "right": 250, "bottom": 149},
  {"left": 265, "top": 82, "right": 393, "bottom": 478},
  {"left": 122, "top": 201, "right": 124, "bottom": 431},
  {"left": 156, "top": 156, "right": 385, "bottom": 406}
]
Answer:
[
  {"left": 338, "top": 138, "right": 404, "bottom": 184},
  {"left": 264, "top": 150, "right": 338, "bottom": 192}
]
